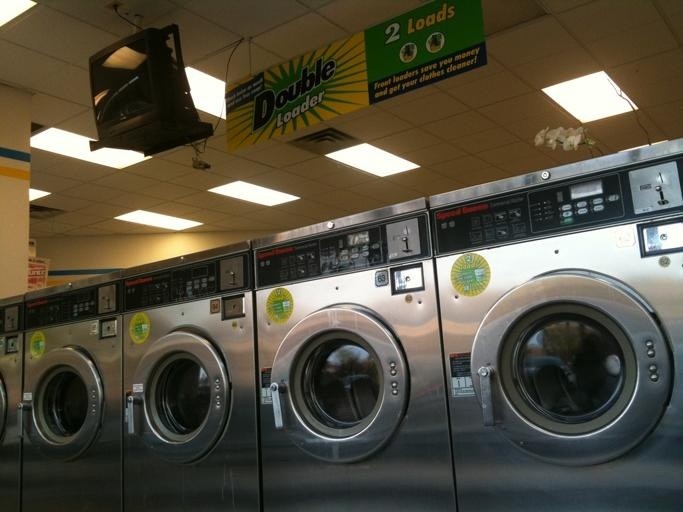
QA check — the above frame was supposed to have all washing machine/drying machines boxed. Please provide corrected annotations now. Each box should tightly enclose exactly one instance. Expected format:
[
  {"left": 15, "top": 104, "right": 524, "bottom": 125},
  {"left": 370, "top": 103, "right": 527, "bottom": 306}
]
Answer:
[
  {"left": 424, "top": 137, "right": 683, "bottom": 511},
  {"left": 125, "top": 240, "right": 264, "bottom": 512},
  {"left": 1, "top": 295, "right": 21, "bottom": 512},
  {"left": 252, "top": 191, "right": 457, "bottom": 511},
  {"left": 22, "top": 269, "right": 129, "bottom": 510}
]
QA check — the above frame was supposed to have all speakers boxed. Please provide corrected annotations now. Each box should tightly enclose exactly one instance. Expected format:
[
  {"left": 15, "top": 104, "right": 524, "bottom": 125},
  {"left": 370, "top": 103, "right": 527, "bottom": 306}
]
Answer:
[{"left": 88, "top": 27, "right": 180, "bottom": 138}]
